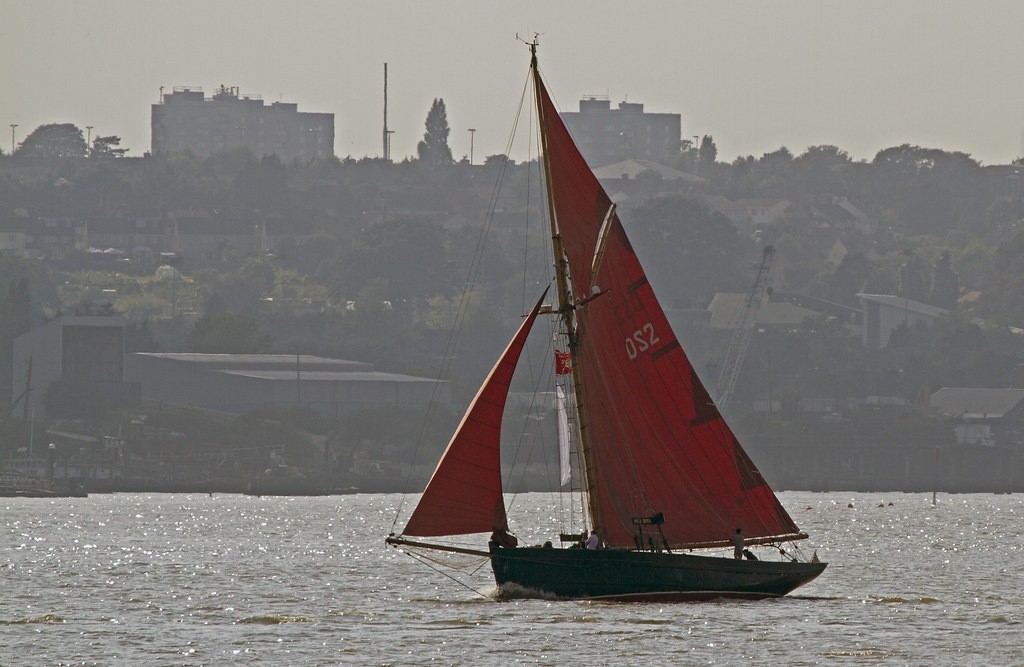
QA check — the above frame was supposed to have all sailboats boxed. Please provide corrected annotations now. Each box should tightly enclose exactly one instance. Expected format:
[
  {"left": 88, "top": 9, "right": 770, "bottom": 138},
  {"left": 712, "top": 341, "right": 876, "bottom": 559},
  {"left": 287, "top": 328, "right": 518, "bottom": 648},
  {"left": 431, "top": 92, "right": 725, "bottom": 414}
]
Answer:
[{"left": 382, "top": 35, "right": 829, "bottom": 602}]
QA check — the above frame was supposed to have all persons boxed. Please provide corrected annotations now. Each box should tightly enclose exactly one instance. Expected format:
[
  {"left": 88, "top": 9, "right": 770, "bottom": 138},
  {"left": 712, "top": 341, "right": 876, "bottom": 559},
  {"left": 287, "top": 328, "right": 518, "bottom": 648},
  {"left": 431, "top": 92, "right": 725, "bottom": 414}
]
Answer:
[
  {"left": 731, "top": 528, "right": 745, "bottom": 560},
  {"left": 585, "top": 531, "right": 598, "bottom": 551},
  {"left": 742, "top": 548, "right": 758, "bottom": 561}
]
